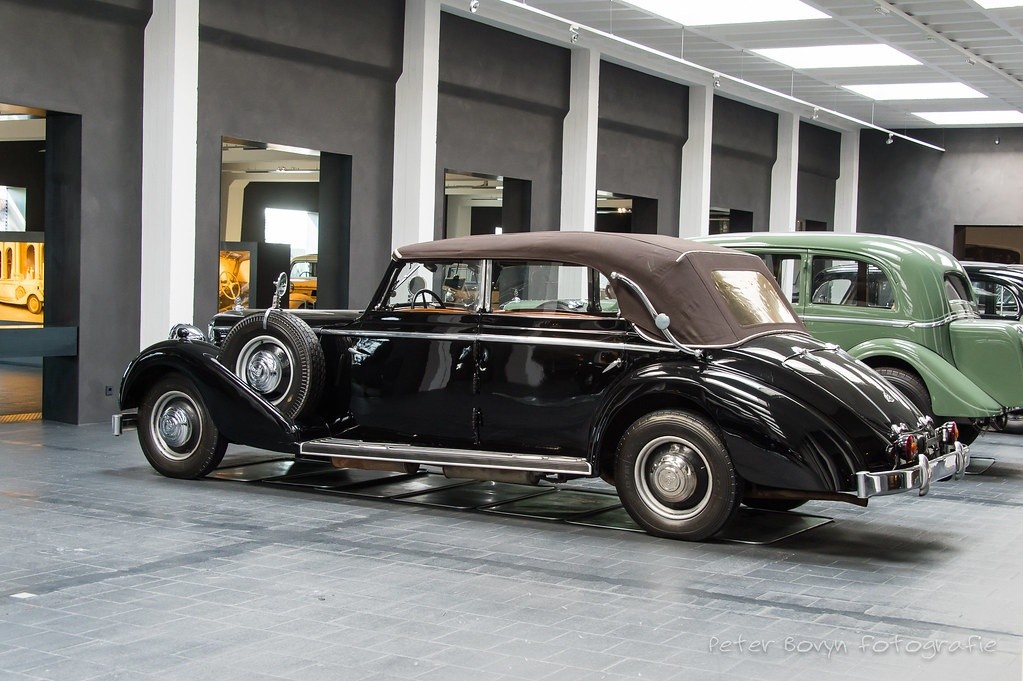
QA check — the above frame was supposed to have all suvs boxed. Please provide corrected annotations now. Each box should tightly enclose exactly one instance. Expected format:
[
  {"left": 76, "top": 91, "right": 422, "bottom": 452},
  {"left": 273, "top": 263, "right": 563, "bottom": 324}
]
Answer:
[
  {"left": 111, "top": 232, "right": 971, "bottom": 544},
  {"left": 500, "top": 230, "right": 1023, "bottom": 447}
]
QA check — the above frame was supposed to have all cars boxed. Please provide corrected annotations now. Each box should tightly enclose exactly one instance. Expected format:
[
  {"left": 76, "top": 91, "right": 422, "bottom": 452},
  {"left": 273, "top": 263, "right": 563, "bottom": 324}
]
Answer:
[{"left": 289, "top": 253, "right": 319, "bottom": 310}]
